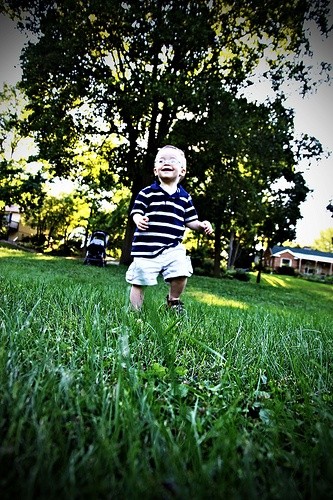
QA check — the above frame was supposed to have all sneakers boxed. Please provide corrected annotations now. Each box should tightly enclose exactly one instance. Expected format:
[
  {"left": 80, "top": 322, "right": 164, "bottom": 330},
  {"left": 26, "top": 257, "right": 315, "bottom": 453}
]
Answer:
[{"left": 165, "top": 295, "right": 185, "bottom": 316}]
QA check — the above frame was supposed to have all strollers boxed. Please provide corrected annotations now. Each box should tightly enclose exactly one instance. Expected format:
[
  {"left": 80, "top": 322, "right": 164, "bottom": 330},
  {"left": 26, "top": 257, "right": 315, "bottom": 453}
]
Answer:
[{"left": 83, "top": 231, "right": 107, "bottom": 266}]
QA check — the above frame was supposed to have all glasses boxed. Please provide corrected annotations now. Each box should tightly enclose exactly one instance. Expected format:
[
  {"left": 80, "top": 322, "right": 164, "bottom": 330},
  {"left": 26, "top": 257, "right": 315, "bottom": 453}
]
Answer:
[{"left": 155, "top": 158, "right": 182, "bottom": 167}]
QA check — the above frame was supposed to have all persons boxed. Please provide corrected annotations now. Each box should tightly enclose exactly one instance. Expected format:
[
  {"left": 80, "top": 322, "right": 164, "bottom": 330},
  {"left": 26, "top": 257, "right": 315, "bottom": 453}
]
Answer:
[{"left": 126, "top": 144, "right": 213, "bottom": 322}]
